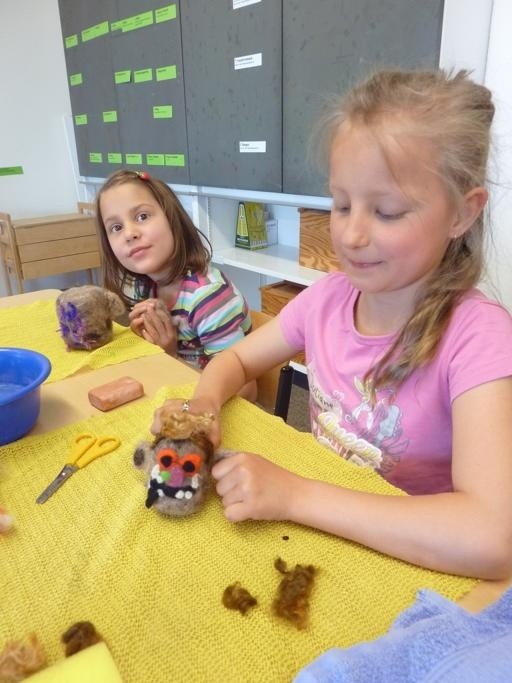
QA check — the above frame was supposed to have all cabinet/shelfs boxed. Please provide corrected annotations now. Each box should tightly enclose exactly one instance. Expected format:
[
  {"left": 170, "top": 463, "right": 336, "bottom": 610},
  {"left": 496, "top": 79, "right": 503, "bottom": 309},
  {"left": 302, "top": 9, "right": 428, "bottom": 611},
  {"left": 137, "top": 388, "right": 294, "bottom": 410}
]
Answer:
[{"left": 1, "top": 200, "right": 107, "bottom": 292}]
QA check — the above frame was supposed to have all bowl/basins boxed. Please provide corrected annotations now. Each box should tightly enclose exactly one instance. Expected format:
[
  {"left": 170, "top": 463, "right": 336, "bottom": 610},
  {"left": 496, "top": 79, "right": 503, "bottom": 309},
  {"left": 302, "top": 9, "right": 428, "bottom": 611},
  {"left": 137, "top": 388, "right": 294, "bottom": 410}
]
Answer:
[{"left": 1, "top": 347, "right": 52, "bottom": 446}]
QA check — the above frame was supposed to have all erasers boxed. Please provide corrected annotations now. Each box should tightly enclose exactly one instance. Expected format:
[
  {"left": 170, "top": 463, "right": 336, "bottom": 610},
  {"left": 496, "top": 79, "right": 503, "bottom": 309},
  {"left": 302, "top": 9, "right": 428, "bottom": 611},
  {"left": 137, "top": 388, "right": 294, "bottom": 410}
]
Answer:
[{"left": 87, "top": 376, "right": 144, "bottom": 411}]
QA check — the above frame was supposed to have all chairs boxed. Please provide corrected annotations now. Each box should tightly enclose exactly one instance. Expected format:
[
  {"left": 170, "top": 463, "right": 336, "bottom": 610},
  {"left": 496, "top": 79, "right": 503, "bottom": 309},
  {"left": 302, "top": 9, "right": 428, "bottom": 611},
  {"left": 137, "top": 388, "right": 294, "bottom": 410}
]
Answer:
[{"left": 239, "top": 306, "right": 294, "bottom": 423}]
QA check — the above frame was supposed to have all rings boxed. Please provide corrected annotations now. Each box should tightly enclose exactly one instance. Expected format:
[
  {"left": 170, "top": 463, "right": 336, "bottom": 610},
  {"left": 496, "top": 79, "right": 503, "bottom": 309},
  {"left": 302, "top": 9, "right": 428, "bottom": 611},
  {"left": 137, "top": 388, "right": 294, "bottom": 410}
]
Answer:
[{"left": 181, "top": 397, "right": 192, "bottom": 412}]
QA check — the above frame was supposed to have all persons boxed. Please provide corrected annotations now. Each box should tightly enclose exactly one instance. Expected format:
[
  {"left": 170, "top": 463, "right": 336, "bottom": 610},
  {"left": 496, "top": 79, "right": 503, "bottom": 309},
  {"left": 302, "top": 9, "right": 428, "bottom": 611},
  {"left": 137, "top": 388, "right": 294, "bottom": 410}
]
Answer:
[
  {"left": 93, "top": 166, "right": 261, "bottom": 405},
  {"left": 143, "top": 62, "right": 512, "bottom": 583}
]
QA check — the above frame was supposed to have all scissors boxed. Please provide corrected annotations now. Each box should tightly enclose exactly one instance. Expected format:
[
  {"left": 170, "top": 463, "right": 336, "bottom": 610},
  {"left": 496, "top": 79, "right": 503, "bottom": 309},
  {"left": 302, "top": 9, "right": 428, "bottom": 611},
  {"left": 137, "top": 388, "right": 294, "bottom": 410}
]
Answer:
[{"left": 36, "top": 433, "right": 121, "bottom": 504}]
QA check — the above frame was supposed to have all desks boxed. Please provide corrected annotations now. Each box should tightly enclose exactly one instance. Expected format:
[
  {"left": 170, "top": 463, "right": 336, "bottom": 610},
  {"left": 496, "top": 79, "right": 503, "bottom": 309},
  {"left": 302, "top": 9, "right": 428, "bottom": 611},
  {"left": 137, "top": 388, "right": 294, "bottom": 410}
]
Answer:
[{"left": 2, "top": 284, "right": 510, "bottom": 677}]
